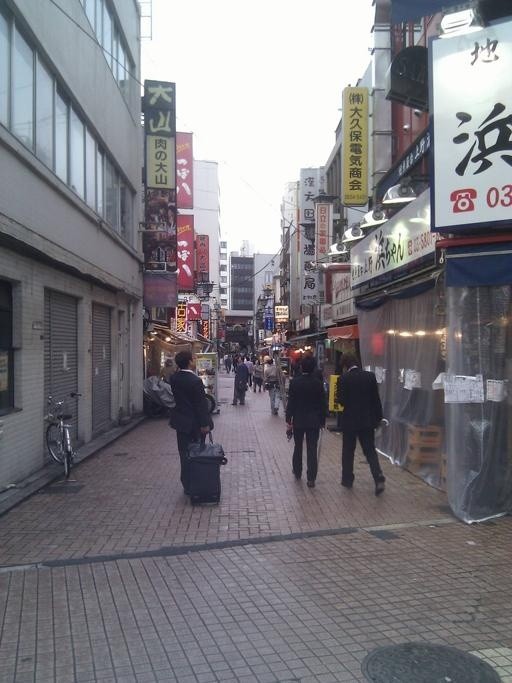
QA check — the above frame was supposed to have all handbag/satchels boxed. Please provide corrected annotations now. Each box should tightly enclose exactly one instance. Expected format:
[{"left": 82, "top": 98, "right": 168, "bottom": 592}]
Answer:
[{"left": 184, "top": 441, "right": 225, "bottom": 463}]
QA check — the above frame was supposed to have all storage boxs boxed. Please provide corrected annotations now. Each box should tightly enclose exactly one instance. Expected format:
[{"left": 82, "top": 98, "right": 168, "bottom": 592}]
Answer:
[
  {"left": 450, "top": 463, "right": 451, "bottom": 466},
  {"left": 440, "top": 453, "right": 448, "bottom": 472},
  {"left": 440, "top": 470, "right": 449, "bottom": 491},
  {"left": 407, "top": 422, "right": 444, "bottom": 442},
  {"left": 405, "top": 439, "right": 444, "bottom": 460},
  {"left": 404, "top": 455, "right": 446, "bottom": 477}
]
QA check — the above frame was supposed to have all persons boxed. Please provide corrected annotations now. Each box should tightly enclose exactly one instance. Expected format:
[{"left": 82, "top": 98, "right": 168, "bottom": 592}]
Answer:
[
  {"left": 261, "top": 356, "right": 283, "bottom": 416},
  {"left": 167, "top": 349, "right": 215, "bottom": 497},
  {"left": 222, "top": 351, "right": 263, "bottom": 406},
  {"left": 335, "top": 355, "right": 386, "bottom": 494},
  {"left": 285, "top": 356, "right": 324, "bottom": 487}
]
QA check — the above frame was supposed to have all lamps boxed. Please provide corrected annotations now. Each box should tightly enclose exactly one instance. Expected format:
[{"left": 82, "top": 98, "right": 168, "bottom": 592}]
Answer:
[
  {"left": 381, "top": 173, "right": 432, "bottom": 203},
  {"left": 341, "top": 222, "right": 366, "bottom": 242},
  {"left": 328, "top": 240, "right": 349, "bottom": 255},
  {"left": 359, "top": 204, "right": 407, "bottom": 228}
]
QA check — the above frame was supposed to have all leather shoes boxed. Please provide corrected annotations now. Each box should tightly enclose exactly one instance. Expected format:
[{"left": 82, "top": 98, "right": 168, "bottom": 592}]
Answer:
[{"left": 375, "top": 474, "right": 385, "bottom": 497}]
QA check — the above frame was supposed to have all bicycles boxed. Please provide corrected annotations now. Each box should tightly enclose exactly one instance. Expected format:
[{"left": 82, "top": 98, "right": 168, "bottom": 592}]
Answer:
[{"left": 45, "top": 394, "right": 82, "bottom": 478}]
